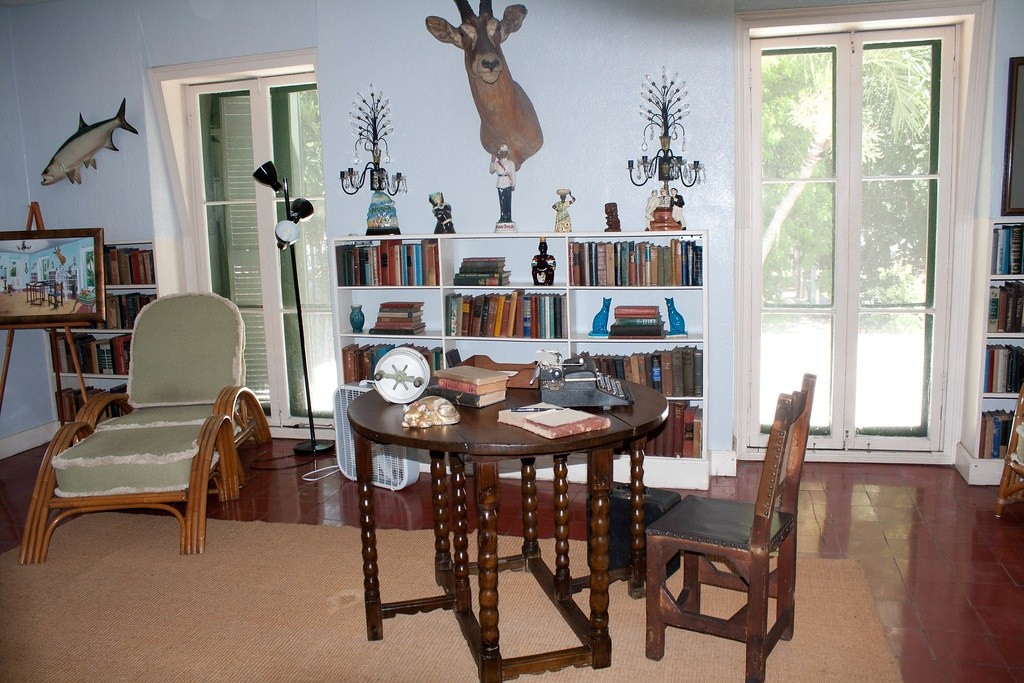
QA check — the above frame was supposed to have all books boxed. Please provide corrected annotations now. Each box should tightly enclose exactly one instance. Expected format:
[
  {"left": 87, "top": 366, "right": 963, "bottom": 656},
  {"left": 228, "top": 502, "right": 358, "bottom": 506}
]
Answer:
[
  {"left": 580, "top": 345, "right": 705, "bottom": 458},
  {"left": 437, "top": 378, "right": 509, "bottom": 395},
  {"left": 433, "top": 366, "right": 511, "bottom": 386},
  {"left": 421, "top": 387, "right": 507, "bottom": 407},
  {"left": 56, "top": 247, "right": 156, "bottom": 424},
  {"left": 369, "top": 301, "right": 426, "bottom": 335},
  {"left": 569, "top": 237, "right": 703, "bottom": 285},
  {"left": 608, "top": 305, "right": 667, "bottom": 339},
  {"left": 336, "top": 240, "right": 440, "bottom": 287},
  {"left": 497, "top": 401, "right": 610, "bottom": 439},
  {"left": 342, "top": 343, "right": 443, "bottom": 384},
  {"left": 446, "top": 289, "right": 569, "bottom": 339},
  {"left": 979, "top": 224, "right": 1024, "bottom": 458},
  {"left": 453, "top": 257, "right": 511, "bottom": 286}
]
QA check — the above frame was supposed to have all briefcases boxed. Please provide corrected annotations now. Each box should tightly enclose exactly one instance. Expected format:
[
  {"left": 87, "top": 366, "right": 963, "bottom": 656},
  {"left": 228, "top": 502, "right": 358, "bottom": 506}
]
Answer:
[{"left": 585, "top": 482, "right": 681, "bottom": 581}]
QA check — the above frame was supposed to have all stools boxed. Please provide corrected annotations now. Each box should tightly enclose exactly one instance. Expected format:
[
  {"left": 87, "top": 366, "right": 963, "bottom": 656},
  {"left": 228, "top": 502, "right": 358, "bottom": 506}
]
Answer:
[{"left": 19, "top": 415, "right": 237, "bottom": 567}]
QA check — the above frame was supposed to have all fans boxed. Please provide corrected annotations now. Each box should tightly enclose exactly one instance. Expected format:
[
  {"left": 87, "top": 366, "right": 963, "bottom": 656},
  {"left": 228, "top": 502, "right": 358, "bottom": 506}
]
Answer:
[{"left": 335, "top": 380, "right": 419, "bottom": 489}]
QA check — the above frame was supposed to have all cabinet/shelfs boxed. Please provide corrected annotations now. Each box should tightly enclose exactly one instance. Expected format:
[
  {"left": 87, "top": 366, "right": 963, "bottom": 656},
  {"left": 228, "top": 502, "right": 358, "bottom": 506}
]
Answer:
[
  {"left": 41, "top": 239, "right": 156, "bottom": 425},
  {"left": 954, "top": 218, "right": 1024, "bottom": 486},
  {"left": 329, "top": 229, "right": 712, "bottom": 492}
]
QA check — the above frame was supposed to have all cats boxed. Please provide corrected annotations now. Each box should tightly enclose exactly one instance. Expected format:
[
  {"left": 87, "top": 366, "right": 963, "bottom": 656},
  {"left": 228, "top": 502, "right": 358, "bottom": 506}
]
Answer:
[{"left": 401, "top": 395, "right": 461, "bottom": 429}]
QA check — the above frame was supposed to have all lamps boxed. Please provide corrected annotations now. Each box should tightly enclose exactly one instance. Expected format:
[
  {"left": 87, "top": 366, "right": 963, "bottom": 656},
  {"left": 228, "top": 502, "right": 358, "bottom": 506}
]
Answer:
[
  {"left": 250, "top": 160, "right": 335, "bottom": 454},
  {"left": 338, "top": 82, "right": 409, "bottom": 237},
  {"left": 626, "top": 65, "right": 706, "bottom": 229}
]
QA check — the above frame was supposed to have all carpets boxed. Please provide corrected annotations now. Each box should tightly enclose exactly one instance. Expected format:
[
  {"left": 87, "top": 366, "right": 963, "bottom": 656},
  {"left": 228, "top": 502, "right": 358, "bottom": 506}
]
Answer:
[{"left": 0, "top": 514, "right": 903, "bottom": 683}]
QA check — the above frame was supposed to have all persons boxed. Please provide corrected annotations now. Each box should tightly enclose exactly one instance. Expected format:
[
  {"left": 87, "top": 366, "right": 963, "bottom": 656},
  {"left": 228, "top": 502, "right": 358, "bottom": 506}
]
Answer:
[
  {"left": 428, "top": 192, "right": 456, "bottom": 234},
  {"left": 552, "top": 189, "right": 577, "bottom": 233},
  {"left": 489, "top": 145, "right": 516, "bottom": 223},
  {"left": 644, "top": 187, "right": 687, "bottom": 230}
]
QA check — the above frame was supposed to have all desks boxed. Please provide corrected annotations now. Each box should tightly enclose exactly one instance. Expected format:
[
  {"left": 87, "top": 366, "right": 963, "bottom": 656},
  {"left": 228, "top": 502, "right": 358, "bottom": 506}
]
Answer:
[{"left": 347, "top": 372, "right": 668, "bottom": 683}]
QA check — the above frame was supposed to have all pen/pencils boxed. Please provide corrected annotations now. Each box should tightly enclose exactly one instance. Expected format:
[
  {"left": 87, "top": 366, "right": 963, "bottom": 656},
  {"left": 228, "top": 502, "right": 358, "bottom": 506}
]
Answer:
[{"left": 512, "top": 407, "right": 564, "bottom": 412}]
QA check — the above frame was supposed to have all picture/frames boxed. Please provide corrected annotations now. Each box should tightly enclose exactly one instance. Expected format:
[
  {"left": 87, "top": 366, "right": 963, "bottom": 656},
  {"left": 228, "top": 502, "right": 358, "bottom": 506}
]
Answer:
[
  {"left": 0, "top": 228, "right": 108, "bottom": 325},
  {"left": 1000, "top": 56, "right": 1024, "bottom": 218}
]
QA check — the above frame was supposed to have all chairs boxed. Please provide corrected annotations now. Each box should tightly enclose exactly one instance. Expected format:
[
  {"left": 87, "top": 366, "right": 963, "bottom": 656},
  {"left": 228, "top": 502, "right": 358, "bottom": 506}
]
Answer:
[
  {"left": 75, "top": 293, "right": 273, "bottom": 487},
  {"left": 641, "top": 373, "right": 816, "bottom": 682}
]
